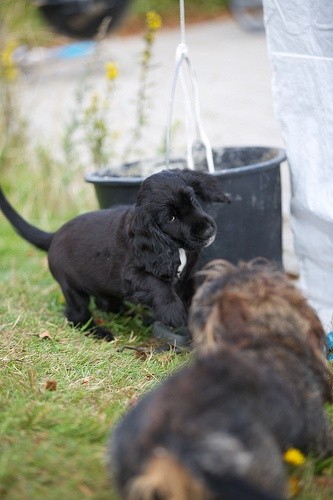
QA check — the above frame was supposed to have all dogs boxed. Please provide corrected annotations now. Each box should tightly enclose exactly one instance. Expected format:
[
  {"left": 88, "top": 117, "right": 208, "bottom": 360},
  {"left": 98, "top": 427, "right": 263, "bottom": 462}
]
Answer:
[
  {"left": 111, "top": 257, "right": 331, "bottom": 500},
  {"left": 1, "top": 170, "right": 232, "bottom": 352}
]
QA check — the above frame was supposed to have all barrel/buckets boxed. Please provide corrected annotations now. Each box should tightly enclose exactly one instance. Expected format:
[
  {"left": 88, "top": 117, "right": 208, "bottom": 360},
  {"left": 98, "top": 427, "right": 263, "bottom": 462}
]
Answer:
[{"left": 82, "top": 53, "right": 288, "bottom": 282}]
